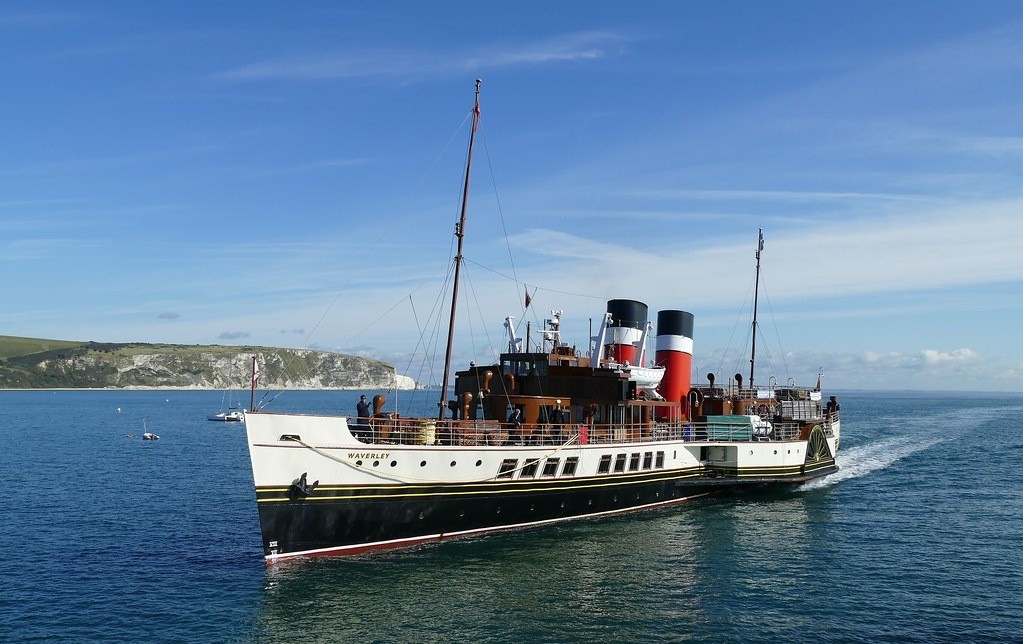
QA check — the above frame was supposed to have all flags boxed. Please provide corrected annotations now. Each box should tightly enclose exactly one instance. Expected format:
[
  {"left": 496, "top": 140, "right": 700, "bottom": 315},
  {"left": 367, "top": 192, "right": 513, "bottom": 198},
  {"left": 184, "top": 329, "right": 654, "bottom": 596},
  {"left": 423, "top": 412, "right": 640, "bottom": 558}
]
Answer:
[
  {"left": 525, "top": 291, "right": 530, "bottom": 308},
  {"left": 254, "top": 362, "right": 260, "bottom": 387}
]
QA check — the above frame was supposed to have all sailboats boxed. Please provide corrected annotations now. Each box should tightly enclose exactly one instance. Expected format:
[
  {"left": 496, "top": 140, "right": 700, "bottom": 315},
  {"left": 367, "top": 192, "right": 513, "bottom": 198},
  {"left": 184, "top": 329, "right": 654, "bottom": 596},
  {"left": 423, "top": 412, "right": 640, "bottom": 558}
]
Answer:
[
  {"left": 241, "top": 77, "right": 711, "bottom": 568},
  {"left": 679, "top": 225, "right": 841, "bottom": 488},
  {"left": 206, "top": 355, "right": 244, "bottom": 422}
]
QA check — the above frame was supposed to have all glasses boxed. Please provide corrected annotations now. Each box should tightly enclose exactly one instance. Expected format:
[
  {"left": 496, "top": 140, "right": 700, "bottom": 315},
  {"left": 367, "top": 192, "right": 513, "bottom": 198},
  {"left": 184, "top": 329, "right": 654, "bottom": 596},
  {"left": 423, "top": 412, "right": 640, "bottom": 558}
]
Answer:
[{"left": 361, "top": 398, "right": 366, "bottom": 400}]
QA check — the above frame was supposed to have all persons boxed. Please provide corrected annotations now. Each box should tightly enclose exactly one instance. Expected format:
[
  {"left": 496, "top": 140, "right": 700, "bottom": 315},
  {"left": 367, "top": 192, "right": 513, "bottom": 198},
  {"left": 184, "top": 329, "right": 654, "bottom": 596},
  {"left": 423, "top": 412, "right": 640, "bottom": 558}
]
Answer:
[
  {"left": 507, "top": 408, "right": 520, "bottom": 444},
  {"left": 356, "top": 395, "right": 372, "bottom": 443},
  {"left": 549, "top": 408, "right": 564, "bottom": 445},
  {"left": 773, "top": 410, "right": 782, "bottom": 441}
]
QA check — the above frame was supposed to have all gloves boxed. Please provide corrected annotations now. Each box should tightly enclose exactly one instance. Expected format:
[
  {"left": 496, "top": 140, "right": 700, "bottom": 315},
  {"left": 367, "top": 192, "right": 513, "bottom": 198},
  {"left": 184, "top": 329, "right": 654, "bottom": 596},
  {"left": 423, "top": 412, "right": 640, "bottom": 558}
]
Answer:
[{"left": 368, "top": 401, "right": 372, "bottom": 406}]
